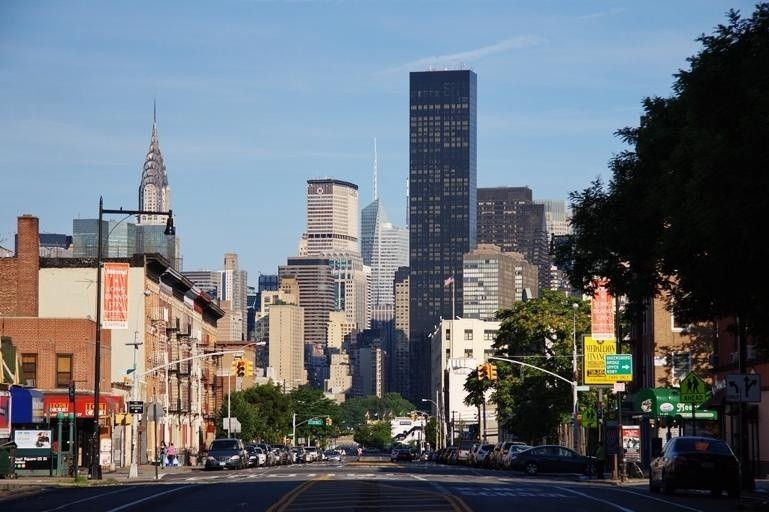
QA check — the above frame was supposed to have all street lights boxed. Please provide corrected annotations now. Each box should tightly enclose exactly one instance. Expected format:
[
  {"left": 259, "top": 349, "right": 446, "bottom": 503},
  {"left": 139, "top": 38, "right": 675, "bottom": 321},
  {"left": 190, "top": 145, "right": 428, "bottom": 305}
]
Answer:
[
  {"left": 69, "top": 412, "right": 77, "bottom": 453},
  {"left": 55, "top": 411, "right": 65, "bottom": 478},
  {"left": 421, "top": 398, "right": 442, "bottom": 449},
  {"left": 571, "top": 303, "right": 580, "bottom": 452},
  {"left": 227, "top": 340, "right": 267, "bottom": 440},
  {"left": 89, "top": 196, "right": 177, "bottom": 479},
  {"left": 453, "top": 364, "right": 487, "bottom": 443}
]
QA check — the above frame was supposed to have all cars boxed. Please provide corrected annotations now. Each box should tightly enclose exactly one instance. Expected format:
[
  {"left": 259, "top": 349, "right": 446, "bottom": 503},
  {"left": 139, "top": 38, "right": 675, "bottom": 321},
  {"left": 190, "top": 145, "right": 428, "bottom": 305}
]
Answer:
[
  {"left": 391, "top": 443, "right": 602, "bottom": 478},
  {"left": 205, "top": 438, "right": 360, "bottom": 471},
  {"left": 648, "top": 437, "right": 744, "bottom": 499},
  {"left": 364, "top": 446, "right": 381, "bottom": 453}
]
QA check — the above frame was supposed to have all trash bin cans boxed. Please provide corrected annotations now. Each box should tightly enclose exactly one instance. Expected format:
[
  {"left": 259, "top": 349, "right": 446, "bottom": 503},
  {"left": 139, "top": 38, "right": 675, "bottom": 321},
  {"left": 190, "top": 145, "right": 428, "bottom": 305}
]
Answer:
[{"left": 0, "top": 442, "right": 17, "bottom": 479}]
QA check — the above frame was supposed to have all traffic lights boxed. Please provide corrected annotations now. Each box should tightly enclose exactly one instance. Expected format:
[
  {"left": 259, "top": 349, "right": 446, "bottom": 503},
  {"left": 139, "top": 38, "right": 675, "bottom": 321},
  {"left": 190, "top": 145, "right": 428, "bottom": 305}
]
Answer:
[
  {"left": 329, "top": 419, "right": 333, "bottom": 425},
  {"left": 485, "top": 363, "right": 491, "bottom": 380},
  {"left": 246, "top": 360, "right": 254, "bottom": 377},
  {"left": 238, "top": 360, "right": 246, "bottom": 378},
  {"left": 231, "top": 359, "right": 237, "bottom": 376},
  {"left": 489, "top": 364, "right": 498, "bottom": 380},
  {"left": 477, "top": 365, "right": 485, "bottom": 382},
  {"left": 410, "top": 412, "right": 418, "bottom": 421},
  {"left": 326, "top": 417, "right": 329, "bottom": 426}
]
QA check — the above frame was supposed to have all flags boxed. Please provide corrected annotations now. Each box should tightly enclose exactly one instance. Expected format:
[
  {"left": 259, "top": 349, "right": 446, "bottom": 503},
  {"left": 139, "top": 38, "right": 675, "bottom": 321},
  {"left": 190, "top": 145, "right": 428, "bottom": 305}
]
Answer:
[{"left": 445, "top": 274, "right": 454, "bottom": 286}]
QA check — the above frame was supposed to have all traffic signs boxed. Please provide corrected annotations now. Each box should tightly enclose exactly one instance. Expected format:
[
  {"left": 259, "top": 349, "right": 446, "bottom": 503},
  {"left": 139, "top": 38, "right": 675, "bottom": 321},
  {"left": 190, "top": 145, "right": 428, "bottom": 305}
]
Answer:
[
  {"left": 128, "top": 400, "right": 144, "bottom": 413},
  {"left": 605, "top": 354, "right": 632, "bottom": 374},
  {"left": 308, "top": 420, "right": 322, "bottom": 425}
]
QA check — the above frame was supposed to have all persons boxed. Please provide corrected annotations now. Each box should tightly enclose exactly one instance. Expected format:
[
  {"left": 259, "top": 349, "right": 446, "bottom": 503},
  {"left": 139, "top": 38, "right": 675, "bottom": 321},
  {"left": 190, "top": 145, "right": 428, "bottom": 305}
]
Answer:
[
  {"left": 166, "top": 442, "right": 176, "bottom": 467},
  {"left": 356, "top": 447, "right": 362, "bottom": 462},
  {"left": 35, "top": 432, "right": 43, "bottom": 447},
  {"left": 43, "top": 433, "right": 50, "bottom": 443},
  {"left": 160, "top": 441, "right": 165, "bottom": 465},
  {"left": 425, "top": 440, "right": 431, "bottom": 451}
]
